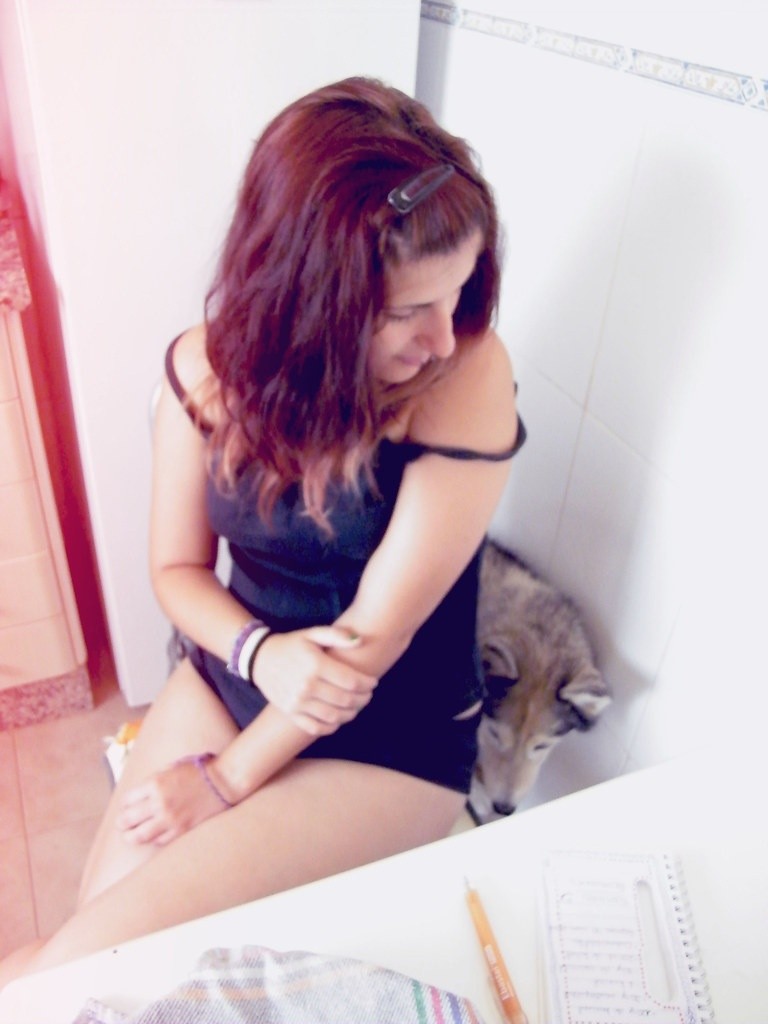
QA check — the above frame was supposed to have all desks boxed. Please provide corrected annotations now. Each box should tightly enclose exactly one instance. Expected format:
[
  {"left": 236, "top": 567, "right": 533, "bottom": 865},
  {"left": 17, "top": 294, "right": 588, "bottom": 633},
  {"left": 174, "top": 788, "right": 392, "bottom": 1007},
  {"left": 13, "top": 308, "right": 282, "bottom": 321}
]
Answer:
[{"left": 0, "top": 748, "right": 765, "bottom": 1023}]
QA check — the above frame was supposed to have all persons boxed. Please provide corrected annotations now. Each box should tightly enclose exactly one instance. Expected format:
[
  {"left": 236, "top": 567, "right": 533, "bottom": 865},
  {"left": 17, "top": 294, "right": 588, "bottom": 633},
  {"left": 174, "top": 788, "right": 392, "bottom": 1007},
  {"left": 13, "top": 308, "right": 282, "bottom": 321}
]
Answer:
[{"left": 3, "top": 77, "right": 532, "bottom": 987}]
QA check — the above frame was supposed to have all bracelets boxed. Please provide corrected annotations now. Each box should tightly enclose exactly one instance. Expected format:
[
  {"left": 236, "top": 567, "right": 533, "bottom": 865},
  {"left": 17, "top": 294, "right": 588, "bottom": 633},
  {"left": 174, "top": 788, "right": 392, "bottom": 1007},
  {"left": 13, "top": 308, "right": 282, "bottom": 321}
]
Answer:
[
  {"left": 176, "top": 747, "right": 236, "bottom": 810},
  {"left": 248, "top": 629, "right": 275, "bottom": 690},
  {"left": 227, "top": 618, "right": 267, "bottom": 680}
]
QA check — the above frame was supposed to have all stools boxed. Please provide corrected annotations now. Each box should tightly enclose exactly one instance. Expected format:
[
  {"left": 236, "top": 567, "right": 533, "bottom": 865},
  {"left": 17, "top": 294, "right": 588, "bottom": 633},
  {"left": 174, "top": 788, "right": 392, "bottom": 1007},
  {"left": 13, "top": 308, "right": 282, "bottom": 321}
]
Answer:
[{"left": 103, "top": 715, "right": 486, "bottom": 839}]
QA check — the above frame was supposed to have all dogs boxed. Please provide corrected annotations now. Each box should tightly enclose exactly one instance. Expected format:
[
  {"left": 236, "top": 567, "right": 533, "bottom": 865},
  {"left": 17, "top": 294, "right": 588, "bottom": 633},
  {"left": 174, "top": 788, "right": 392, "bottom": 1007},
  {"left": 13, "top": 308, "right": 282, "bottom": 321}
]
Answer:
[{"left": 164, "top": 529, "right": 615, "bottom": 816}]
